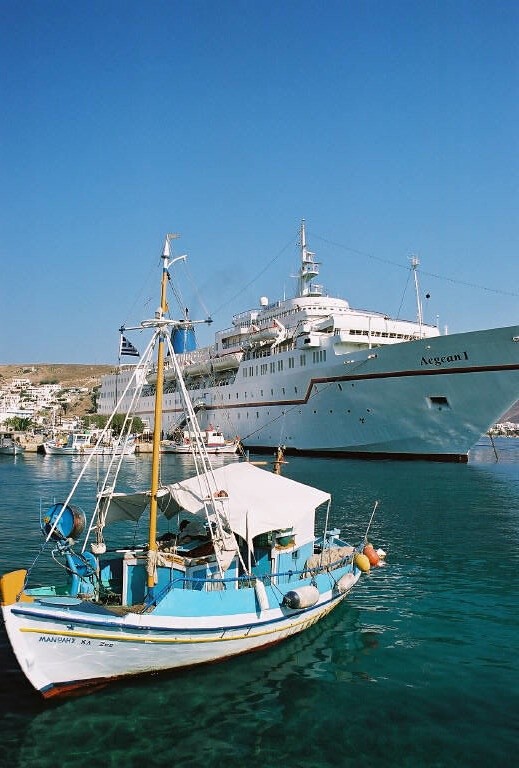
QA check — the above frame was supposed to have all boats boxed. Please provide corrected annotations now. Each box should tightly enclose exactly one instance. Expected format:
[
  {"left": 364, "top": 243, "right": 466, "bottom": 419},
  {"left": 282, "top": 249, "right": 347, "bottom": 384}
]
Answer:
[
  {"left": 0, "top": 409, "right": 240, "bottom": 455},
  {"left": 145, "top": 351, "right": 246, "bottom": 384},
  {"left": 248, "top": 327, "right": 277, "bottom": 342},
  {"left": 0, "top": 230, "right": 378, "bottom": 702},
  {"left": 96, "top": 219, "right": 519, "bottom": 464}
]
